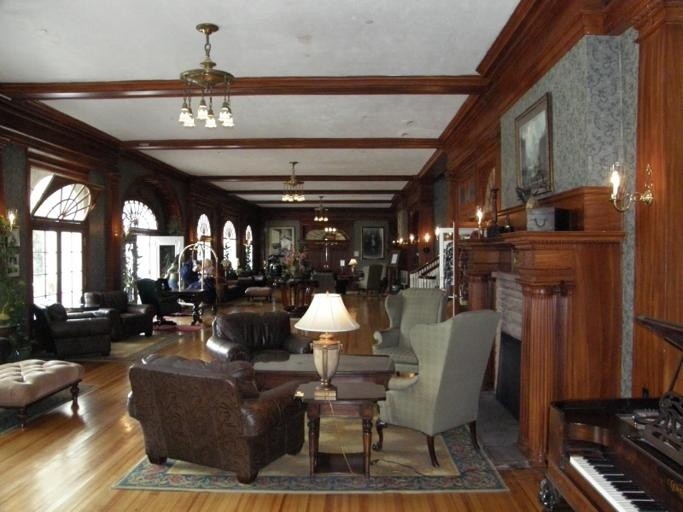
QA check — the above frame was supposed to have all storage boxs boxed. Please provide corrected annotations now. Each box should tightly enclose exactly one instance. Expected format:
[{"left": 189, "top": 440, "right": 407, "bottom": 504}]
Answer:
[{"left": 524, "top": 205, "right": 558, "bottom": 233}]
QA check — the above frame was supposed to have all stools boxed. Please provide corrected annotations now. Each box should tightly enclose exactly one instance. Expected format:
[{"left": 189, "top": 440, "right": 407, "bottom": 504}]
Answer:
[
  {"left": 244, "top": 287, "right": 272, "bottom": 302},
  {"left": 0, "top": 359, "right": 84, "bottom": 432}
]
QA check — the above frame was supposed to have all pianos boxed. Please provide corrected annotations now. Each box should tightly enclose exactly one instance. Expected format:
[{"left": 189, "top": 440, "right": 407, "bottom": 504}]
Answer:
[{"left": 541, "top": 316, "right": 682, "bottom": 511}]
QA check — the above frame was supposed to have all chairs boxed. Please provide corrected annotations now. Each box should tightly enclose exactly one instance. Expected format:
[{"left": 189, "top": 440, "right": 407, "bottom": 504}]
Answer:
[
  {"left": 127, "top": 352, "right": 307, "bottom": 484},
  {"left": 371, "top": 285, "right": 453, "bottom": 378},
  {"left": 367, "top": 309, "right": 499, "bottom": 470},
  {"left": 0, "top": 276, "right": 178, "bottom": 356},
  {"left": 206, "top": 309, "right": 313, "bottom": 391}
]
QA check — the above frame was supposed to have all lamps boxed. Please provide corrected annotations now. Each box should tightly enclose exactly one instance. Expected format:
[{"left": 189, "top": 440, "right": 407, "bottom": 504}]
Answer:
[
  {"left": 293, "top": 291, "right": 360, "bottom": 404},
  {"left": 324, "top": 208, "right": 337, "bottom": 233},
  {"left": 347, "top": 258, "right": 358, "bottom": 273},
  {"left": 605, "top": 157, "right": 657, "bottom": 214},
  {"left": 174, "top": 21, "right": 236, "bottom": 129},
  {"left": 280, "top": 160, "right": 307, "bottom": 204},
  {"left": 312, "top": 195, "right": 329, "bottom": 223}
]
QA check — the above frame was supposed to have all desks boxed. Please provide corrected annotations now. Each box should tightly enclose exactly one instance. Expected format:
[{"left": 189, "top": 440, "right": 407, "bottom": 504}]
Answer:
[
  {"left": 289, "top": 375, "right": 385, "bottom": 484},
  {"left": 173, "top": 289, "right": 207, "bottom": 325}
]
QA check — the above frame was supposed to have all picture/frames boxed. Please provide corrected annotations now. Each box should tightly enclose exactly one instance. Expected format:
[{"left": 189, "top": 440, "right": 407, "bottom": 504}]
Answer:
[
  {"left": 510, "top": 89, "right": 556, "bottom": 198},
  {"left": 361, "top": 226, "right": 385, "bottom": 261},
  {"left": 266, "top": 224, "right": 295, "bottom": 261}
]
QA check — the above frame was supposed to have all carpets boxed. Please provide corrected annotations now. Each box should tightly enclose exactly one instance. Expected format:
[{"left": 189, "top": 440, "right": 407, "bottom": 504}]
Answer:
[
  {"left": 109, "top": 413, "right": 513, "bottom": 495},
  {"left": 0, "top": 380, "right": 97, "bottom": 427}
]
